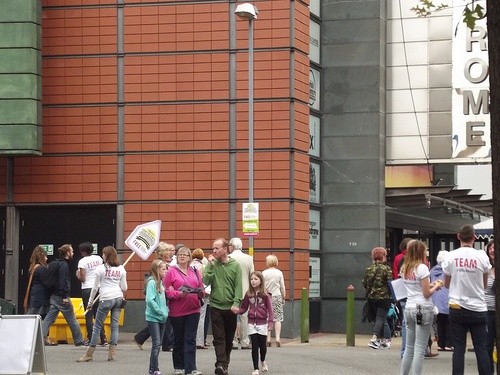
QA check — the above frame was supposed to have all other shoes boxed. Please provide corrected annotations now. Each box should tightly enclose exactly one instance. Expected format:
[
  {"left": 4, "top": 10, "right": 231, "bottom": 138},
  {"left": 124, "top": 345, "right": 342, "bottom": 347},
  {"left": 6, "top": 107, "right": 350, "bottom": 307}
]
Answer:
[
  {"left": 102, "top": 340, "right": 108, "bottom": 347},
  {"left": 197, "top": 346, "right": 208, "bottom": 349},
  {"left": 379, "top": 341, "right": 390, "bottom": 349},
  {"left": 266, "top": 342, "right": 271, "bottom": 347},
  {"left": 174, "top": 369, "right": 186, "bottom": 375},
  {"left": 262, "top": 365, "right": 267, "bottom": 373},
  {"left": 76, "top": 341, "right": 88, "bottom": 346},
  {"left": 152, "top": 371, "right": 162, "bottom": 375},
  {"left": 252, "top": 370, "right": 259, "bottom": 375},
  {"left": 276, "top": 341, "right": 280, "bottom": 347},
  {"left": 438, "top": 347, "right": 443, "bottom": 350},
  {"left": 137, "top": 343, "right": 143, "bottom": 350},
  {"left": 241, "top": 347, "right": 252, "bottom": 349},
  {"left": 443, "top": 347, "right": 452, "bottom": 351},
  {"left": 214, "top": 366, "right": 228, "bottom": 375},
  {"left": 186, "top": 370, "right": 202, "bottom": 375},
  {"left": 232, "top": 347, "right": 238, "bottom": 349},
  {"left": 368, "top": 339, "right": 379, "bottom": 348},
  {"left": 45, "top": 340, "right": 58, "bottom": 346}
]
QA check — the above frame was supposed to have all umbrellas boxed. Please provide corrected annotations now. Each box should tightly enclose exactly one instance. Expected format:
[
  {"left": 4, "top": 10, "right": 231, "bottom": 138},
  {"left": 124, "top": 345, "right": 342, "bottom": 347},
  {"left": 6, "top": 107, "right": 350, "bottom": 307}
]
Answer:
[{"left": 472, "top": 217, "right": 494, "bottom": 242}]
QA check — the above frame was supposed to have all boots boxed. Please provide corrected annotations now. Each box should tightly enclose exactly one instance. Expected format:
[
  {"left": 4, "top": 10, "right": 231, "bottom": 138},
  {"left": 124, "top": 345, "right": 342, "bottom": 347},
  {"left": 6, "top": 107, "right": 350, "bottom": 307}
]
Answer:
[
  {"left": 77, "top": 345, "right": 95, "bottom": 362},
  {"left": 108, "top": 344, "right": 116, "bottom": 361}
]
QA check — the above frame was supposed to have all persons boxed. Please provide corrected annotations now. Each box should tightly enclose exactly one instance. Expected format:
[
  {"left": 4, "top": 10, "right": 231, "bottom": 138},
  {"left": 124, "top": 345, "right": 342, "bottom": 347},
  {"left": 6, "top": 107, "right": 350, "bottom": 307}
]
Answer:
[
  {"left": 393, "top": 238, "right": 409, "bottom": 328},
  {"left": 261, "top": 254, "right": 286, "bottom": 347},
  {"left": 201, "top": 256, "right": 212, "bottom": 346},
  {"left": 189, "top": 248, "right": 208, "bottom": 349},
  {"left": 233, "top": 271, "right": 274, "bottom": 375},
  {"left": 202, "top": 238, "right": 243, "bottom": 375},
  {"left": 76, "top": 242, "right": 108, "bottom": 347},
  {"left": 166, "top": 244, "right": 175, "bottom": 266},
  {"left": 134, "top": 242, "right": 173, "bottom": 351},
  {"left": 145, "top": 259, "right": 169, "bottom": 375},
  {"left": 227, "top": 237, "right": 254, "bottom": 350},
  {"left": 362, "top": 247, "right": 393, "bottom": 350},
  {"left": 26, "top": 246, "right": 47, "bottom": 314},
  {"left": 75, "top": 246, "right": 127, "bottom": 363},
  {"left": 428, "top": 225, "right": 497, "bottom": 375},
  {"left": 399, "top": 241, "right": 444, "bottom": 375},
  {"left": 169, "top": 243, "right": 184, "bottom": 266},
  {"left": 162, "top": 247, "right": 203, "bottom": 375},
  {"left": 41, "top": 244, "right": 88, "bottom": 346}
]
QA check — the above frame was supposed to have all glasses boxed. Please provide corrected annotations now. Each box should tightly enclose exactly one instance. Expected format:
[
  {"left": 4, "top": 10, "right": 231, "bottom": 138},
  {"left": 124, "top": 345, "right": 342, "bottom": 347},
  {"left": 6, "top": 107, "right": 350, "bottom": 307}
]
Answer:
[{"left": 178, "top": 254, "right": 190, "bottom": 257}]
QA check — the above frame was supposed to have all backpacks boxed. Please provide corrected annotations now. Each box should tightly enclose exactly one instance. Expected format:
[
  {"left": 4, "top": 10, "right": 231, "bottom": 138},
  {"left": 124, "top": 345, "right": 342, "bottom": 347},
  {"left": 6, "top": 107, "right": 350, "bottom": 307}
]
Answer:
[{"left": 42, "top": 259, "right": 67, "bottom": 292}]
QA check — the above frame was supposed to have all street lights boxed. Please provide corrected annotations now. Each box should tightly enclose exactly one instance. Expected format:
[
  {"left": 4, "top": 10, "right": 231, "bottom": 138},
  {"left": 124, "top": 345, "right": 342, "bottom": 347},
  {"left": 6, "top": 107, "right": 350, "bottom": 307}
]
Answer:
[{"left": 235, "top": 2, "right": 259, "bottom": 257}]
